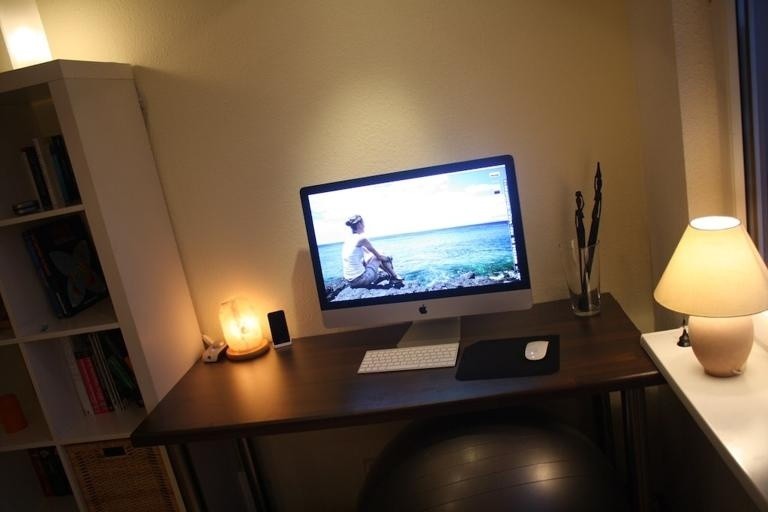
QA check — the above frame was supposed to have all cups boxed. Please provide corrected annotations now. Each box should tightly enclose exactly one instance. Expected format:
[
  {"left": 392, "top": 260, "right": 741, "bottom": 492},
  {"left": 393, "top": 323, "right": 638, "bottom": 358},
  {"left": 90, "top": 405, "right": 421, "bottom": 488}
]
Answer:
[
  {"left": 559, "top": 238, "right": 602, "bottom": 316},
  {"left": 1, "top": 394, "right": 29, "bottom": 433}
]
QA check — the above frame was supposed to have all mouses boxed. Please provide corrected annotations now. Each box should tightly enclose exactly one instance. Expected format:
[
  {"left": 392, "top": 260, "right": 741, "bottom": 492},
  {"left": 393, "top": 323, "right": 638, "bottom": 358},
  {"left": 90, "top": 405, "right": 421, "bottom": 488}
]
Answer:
[{"left": 524, "top": 340, "right": 549, "bottom": 361}]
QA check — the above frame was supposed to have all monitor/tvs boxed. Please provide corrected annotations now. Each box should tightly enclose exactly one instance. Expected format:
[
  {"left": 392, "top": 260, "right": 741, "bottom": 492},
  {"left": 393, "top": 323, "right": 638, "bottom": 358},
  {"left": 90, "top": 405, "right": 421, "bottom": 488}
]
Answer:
[{"left": 299, "top": 154, "right": 533, "bottom": 347}]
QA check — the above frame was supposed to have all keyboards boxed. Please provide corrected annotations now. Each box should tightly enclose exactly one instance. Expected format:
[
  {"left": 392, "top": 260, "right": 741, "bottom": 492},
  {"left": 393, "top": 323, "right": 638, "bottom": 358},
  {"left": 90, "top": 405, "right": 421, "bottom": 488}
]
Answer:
[{"left": 356, "top": 343, "right": 460, "bottom": 374}]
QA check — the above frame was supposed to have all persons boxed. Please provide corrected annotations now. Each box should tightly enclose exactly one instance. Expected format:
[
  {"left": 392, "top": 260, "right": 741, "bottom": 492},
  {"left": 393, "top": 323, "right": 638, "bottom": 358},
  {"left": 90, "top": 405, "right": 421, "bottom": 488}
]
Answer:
[{"left": 340, "top": 214, "right": 403, "bottom": 288}]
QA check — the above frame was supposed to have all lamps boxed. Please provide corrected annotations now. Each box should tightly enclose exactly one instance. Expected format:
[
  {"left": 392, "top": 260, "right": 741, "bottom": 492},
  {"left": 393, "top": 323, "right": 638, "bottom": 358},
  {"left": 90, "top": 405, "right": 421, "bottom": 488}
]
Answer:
[
  {"left": 219, "top": 292, "right": 263, "bottom": 350},
  {"left": 652, "top": 211, "right": 768, "bottom": 378}
]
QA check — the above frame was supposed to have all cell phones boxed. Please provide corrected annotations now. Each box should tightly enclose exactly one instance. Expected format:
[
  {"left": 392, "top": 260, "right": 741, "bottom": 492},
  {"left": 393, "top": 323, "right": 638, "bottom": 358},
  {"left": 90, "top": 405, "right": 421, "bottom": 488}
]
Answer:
[{"left": 268, "top": 310, "right": 290, "bottom": 345}]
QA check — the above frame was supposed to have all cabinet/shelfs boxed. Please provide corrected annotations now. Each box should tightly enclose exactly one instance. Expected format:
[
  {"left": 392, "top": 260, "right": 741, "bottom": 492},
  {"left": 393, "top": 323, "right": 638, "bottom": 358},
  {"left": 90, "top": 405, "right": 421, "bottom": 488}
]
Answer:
[{"left": 1, "top": 58, "right": 251, "bottom": 510}]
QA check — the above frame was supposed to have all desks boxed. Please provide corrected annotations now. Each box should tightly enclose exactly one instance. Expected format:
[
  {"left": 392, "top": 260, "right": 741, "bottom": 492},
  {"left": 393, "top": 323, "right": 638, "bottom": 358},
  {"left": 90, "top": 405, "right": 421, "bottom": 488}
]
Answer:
[
  {"left": 129, "top": 289, "right": 666, "bottom": 511},
  {"left": 640, "top": 313, "right": 765, "bottom": 510}
]
